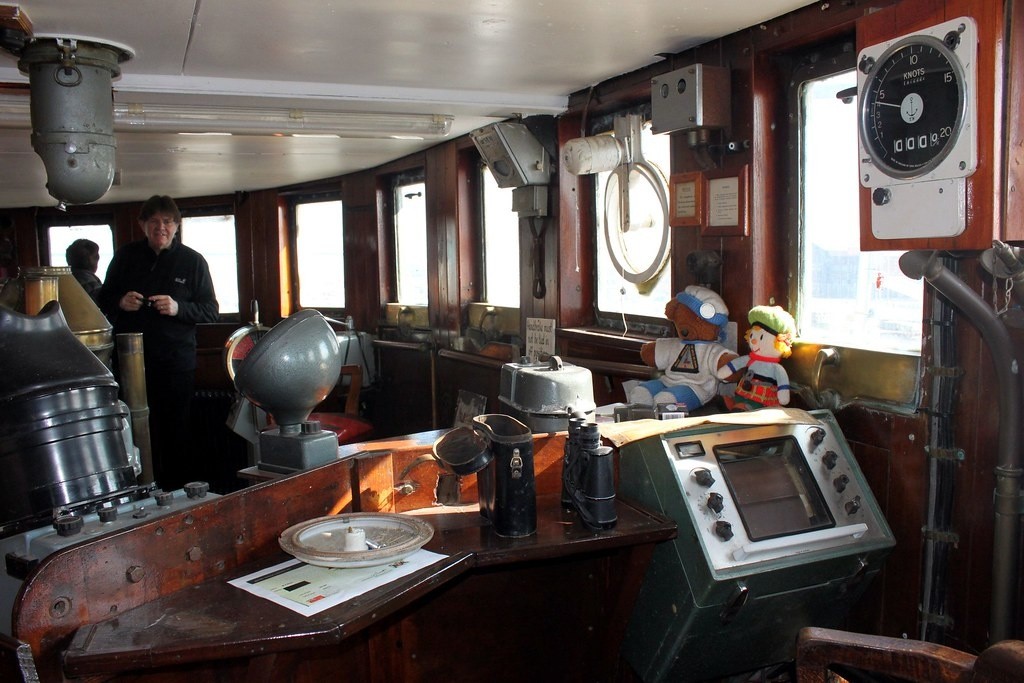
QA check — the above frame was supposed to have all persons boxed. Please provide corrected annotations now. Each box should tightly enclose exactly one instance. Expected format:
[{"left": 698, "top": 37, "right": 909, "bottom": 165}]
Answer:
[
  {"left": 66, "top": 239, "right": 102, "bottom": 303},
  {"left": 96, "top": 196, "right": 219, "bottom": 491}
]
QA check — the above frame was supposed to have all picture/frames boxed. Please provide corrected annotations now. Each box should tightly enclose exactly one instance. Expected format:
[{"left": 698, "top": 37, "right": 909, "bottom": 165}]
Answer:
[
  {"left": 669, "top": 171, "right": 701, "bottom": 227},
  {"left": 701, "top": 164, "right": 751, "bottom": 237}
]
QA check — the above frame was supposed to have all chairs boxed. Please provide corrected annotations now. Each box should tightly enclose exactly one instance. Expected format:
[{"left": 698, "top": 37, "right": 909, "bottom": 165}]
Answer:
[
  {"left": 794, "top": 625, "right": 1024, "bottom": 682},
  {"left": 268, "top": 363, "right": 375, "bottom": 447}
]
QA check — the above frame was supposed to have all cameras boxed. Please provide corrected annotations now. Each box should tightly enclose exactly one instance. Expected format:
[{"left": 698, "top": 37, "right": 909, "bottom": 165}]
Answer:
[{"left": 138, "top": 298, "right": 155, "bottom": 307}]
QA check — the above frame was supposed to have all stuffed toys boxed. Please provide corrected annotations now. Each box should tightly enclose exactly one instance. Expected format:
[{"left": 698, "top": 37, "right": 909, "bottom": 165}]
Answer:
[
  {"left": 629, "top": 285, "right": 746, "bottom": 413},
  {"left": 717, "top": 305, "right": 798, "bottom": 410}
]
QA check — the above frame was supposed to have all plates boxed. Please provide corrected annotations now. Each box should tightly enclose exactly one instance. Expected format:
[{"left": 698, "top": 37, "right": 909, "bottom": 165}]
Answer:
[{"left": 279, "top": 511, "right": 434, "bottom": 568}]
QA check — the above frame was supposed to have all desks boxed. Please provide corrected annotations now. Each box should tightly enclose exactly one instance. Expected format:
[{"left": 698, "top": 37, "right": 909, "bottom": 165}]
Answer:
[{"left": 14, "top": 428, "right": 680, "bottom": 681}]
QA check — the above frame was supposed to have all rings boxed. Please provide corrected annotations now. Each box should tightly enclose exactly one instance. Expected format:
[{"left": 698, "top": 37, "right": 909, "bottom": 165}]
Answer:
[{"left": 161, "top": 305, "right": 163, "bottom": 310}]
